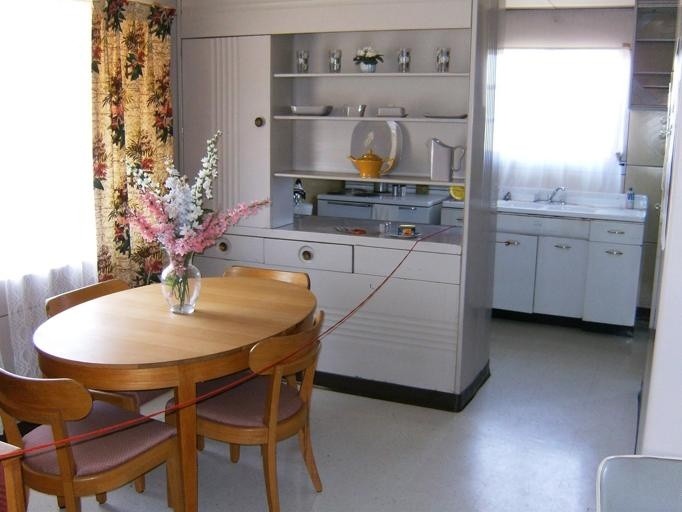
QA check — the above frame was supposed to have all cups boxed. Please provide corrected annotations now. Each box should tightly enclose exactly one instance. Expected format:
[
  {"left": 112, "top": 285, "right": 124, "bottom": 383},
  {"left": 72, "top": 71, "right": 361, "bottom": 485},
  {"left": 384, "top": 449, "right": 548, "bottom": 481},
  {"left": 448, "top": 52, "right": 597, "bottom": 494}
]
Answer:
[
  {"left": 397, "top": 48, "right": 410, "bottom": 73},
  {"left": 296, "top": 49, "right": 309, "bottom": 74},
  {"left": 345, "top": 104, "right": 367, "bottom": 117},
  {"left": 328, "top": 49, "right": 341, "bottom": 73},
  {"left": 374, "top": 181, "right": 427, "bottom": 197},
  {"left": 435, "top": 46, "right": 450, "bottom": 72},
  {"left": 352, "top": 217, "right": 421, "bottom": 240}
]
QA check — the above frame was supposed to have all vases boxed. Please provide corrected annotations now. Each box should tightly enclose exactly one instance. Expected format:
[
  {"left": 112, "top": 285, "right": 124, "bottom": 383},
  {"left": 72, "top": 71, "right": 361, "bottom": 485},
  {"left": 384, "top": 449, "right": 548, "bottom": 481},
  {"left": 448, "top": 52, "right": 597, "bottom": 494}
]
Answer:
[
  {"left": 358, "top": 62, "right": 377, "bottom": 72},
  {"left": 159, "top": 248, "right": 202, "bottom": 314}
]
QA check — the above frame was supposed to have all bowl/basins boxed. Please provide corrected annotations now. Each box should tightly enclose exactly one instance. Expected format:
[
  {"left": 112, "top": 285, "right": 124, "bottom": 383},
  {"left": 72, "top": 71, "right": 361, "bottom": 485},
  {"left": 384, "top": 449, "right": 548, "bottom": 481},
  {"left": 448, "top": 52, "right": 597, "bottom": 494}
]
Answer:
[
  {"left": 449, "top": 187, "right": 464, "bottom": 200},
  {"left": 377, "top": 108, "right": 404, "bottom": 115},
  {"left": 291, "top": 105, "right": 333, "bottom": 115}
]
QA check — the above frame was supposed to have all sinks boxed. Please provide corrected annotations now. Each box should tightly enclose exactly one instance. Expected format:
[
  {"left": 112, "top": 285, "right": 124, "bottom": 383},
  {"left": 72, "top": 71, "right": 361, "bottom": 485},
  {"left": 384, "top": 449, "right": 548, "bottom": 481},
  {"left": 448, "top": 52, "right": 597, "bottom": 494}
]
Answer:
[{"left": 501, "top": 201, "right": 591, "bottom": 213}]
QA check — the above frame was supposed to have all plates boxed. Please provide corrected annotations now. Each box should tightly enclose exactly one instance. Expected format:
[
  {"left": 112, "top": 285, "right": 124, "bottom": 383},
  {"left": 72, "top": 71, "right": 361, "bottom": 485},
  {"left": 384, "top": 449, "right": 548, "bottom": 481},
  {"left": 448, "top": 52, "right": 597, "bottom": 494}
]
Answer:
[
  {"left": 424, "top": 112, "right": 466, "bottom": 118},
  {"left": 351, "top": 121, "right": 402, "bottom": 175},
  {"left": 376, "top": 114, "right": 408, "bottom": 117}
]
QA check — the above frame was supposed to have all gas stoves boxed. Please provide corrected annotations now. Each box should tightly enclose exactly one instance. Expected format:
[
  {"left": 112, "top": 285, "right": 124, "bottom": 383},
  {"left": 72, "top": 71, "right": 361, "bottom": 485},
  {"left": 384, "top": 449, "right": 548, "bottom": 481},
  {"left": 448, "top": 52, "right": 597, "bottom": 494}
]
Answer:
[{"left": 316, "top": 187, "right": 391, "bottom": 203}]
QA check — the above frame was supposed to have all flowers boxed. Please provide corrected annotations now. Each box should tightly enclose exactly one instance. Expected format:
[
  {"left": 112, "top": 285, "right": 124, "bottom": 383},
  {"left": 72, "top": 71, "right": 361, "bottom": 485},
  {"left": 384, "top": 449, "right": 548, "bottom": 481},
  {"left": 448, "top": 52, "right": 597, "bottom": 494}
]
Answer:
[
  {"left": 351, "top": 46, "right": 385, "bottom": 64},
  {"left": 115, "top": 122, "right": 272, "bottom": 310}
]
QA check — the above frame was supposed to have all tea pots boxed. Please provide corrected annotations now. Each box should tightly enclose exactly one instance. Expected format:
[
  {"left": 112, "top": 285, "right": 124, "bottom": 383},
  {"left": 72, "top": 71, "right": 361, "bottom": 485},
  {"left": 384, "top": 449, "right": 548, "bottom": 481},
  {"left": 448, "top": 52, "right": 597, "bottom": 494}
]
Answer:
[
  {"left": 347, "top": 150, "right": 395, "bottom": 178},
  {"left": 431, "top": 138, "right": 465, "bottom": 181}
]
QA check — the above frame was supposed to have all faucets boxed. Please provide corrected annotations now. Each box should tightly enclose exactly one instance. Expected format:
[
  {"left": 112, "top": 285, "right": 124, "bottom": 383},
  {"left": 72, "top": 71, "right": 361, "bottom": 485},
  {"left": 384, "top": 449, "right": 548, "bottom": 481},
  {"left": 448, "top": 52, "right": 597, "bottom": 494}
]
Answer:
[{"left": 548, "top": 187, "right": 565, "bottom": 201}]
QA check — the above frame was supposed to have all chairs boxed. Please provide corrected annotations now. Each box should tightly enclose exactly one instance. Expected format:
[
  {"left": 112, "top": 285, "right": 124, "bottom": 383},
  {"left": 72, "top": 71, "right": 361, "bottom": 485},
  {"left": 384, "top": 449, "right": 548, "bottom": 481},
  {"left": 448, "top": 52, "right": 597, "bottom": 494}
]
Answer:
[
  {"left": 1, "top": 368, "right": 186, "bottom": 512},
  {"left": 42, "top": 279, "right": 195, "bottom": 492},
  {"left": 166, "top": 308, "right": 327, "bottom": 510},
  {"left": 592, "top": 452, "right": 681, "bottom": 511},
  {"left": 196, "top": 264, "right": 312, "bottom": 464}
]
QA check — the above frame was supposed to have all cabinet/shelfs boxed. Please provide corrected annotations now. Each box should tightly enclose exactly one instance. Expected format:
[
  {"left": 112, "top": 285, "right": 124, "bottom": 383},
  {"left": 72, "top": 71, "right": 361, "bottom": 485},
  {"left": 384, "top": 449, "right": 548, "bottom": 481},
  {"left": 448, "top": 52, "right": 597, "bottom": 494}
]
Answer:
[
  {"left": 579, "top": 219, "right": 646, "bottom": 334},
  {"left": 266, "top": 238, "right": 496, "bottom": 410},
  {"left": 271, "top": 0, "right": 501, "bottom": 247},
  {"left": 623, "top": 112, "right": 666, "bottom": 315},
  {"left": 437, "top": 205, "right": 467, "bottom": 227},
  {"left": 177, "top": 3, "right": 294, "bottom": 230},
  {"left": 626, "top": 0, "right": 677, "bottom": 110},
  {"left": 371, "top": 202, "right": 441, "bottom": 226},
  {"left": 493, "top": 212, "right": 588, "bottom": 324},
  {"left": 187, "top": 232, "right": 266, "bottom": 275}
]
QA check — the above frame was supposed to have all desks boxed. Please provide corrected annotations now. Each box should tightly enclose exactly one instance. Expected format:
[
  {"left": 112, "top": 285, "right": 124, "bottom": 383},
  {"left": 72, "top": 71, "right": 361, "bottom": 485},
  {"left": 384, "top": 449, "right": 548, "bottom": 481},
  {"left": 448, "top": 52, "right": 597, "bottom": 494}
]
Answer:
[
  {"left": 32, "top": 274, "right": 318, "bottom": 512},
  {"left": 2, "top": 440, "right": 26, "bottom": 511}
]
